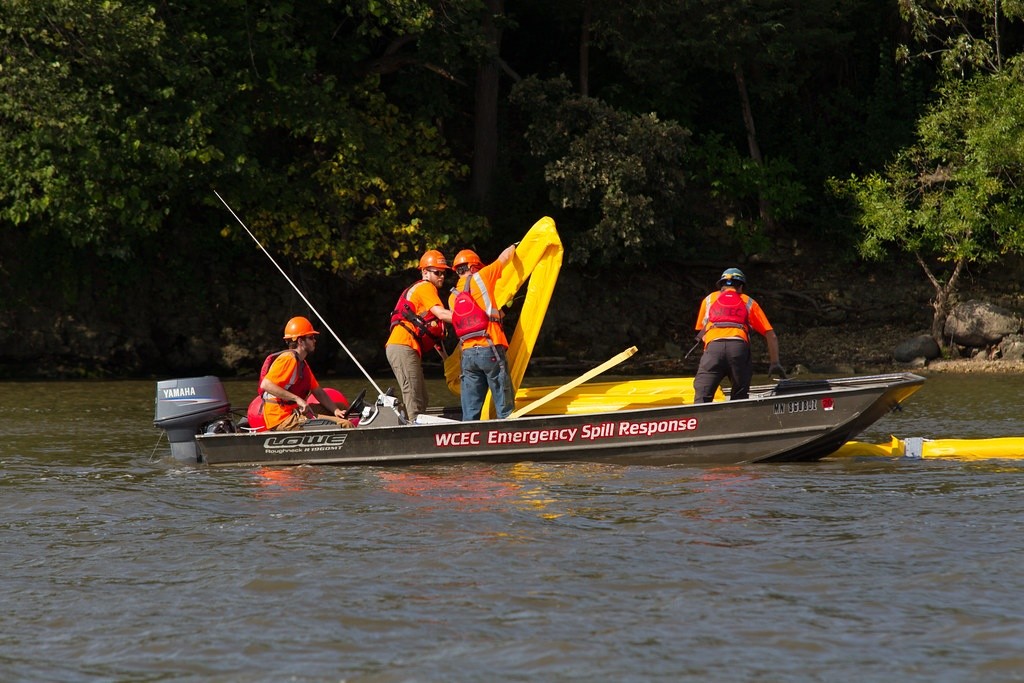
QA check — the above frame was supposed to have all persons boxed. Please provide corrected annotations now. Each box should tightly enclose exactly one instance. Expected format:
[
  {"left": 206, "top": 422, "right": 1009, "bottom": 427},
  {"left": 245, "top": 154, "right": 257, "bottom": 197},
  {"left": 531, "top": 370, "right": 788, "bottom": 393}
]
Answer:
[
  {"left": 260, "top": 316, "right": 356, "bottom": 431},
  {"left": 695, "top": 268, "right": 787, "bottom": 403},
  {"left": 384, "top": 250, "right": 453, "bottom": 422},
  {"left": 448, "top": 241, "right": 520, "bottom": 421}
]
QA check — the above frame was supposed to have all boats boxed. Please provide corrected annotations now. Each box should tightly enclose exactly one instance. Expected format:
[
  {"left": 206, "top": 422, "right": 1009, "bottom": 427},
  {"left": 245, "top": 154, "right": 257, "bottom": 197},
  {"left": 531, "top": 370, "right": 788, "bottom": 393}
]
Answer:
[{"left": 151, "top": 370, "right": 926, "bottom": 469}]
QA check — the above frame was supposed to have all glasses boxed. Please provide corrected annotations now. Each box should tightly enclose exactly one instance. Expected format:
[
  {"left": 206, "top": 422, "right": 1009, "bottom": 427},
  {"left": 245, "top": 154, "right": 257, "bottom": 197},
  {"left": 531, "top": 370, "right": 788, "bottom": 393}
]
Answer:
[
  {"left": 427, "top": 269, "right": 446, "bottom": 276},
  {"left": 305, "top": 336, "right": 315, "bottom": 340}
]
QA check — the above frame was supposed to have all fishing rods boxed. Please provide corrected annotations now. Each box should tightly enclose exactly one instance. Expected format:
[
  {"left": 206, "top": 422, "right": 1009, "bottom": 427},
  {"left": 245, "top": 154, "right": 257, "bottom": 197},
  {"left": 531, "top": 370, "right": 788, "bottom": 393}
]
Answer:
[{"left": 212, "top": 188, "right": 406, "bottom": 425}]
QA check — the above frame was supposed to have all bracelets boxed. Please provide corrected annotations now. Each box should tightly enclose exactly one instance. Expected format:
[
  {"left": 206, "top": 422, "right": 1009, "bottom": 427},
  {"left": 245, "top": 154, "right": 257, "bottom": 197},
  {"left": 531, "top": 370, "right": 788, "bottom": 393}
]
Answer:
[{"left": 512, "top": 243, "right": 517, "bottom": 249}]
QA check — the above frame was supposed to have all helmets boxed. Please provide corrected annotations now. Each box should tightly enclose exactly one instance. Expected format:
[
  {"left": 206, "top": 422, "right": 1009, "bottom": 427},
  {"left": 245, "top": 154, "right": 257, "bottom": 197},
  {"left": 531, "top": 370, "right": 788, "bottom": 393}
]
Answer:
[
  {"left": 417, "top": 250, "right": 451, "bottom": 270},
  {"left": 282, "top": 317, "right": 321, "bottom": 341},
  {"left": 716, "top": 268, "right": 749, "bottom": 293},
  {"left": 452, "top": 250, "right": 487, "bottom": 272}
]
